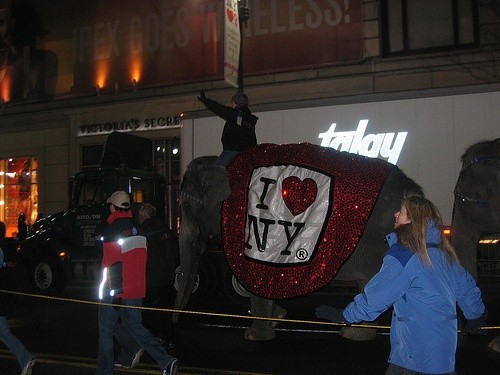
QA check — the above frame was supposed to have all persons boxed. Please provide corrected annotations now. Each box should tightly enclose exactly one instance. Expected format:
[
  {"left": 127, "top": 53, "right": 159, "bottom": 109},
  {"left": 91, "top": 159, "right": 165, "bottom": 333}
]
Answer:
[
  {"left": 197, "top": 90, "right": 259, "bottom": 168},
  {"left": 16, "top": 216, "right": 28, "bottom": 243},
  {"left": 316, "top": 195, "right": 489, "bottom": 375},
  {"left": 96, "top": 190, "right": 178, "bottom": 375},
  {"left": 113, "top": 203, "right": 174, "bottom": 369},
  {"left": 0, "top": 222, "right": 37, "bottom": 375}
]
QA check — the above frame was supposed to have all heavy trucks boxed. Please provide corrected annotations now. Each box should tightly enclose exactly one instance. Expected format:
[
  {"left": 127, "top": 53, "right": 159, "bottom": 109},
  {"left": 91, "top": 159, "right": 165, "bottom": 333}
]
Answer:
[{"left": 15, "top": 82, "right": 500, "bottom": 305}]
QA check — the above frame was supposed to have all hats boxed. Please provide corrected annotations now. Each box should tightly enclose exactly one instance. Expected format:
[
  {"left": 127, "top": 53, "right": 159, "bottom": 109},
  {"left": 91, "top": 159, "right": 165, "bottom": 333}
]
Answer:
[
  {"left": 232, "top": 91, "right": 248, "bottom": 105},
  {"left": 107, "top": 190, "right": 131, "bottom": 208}
]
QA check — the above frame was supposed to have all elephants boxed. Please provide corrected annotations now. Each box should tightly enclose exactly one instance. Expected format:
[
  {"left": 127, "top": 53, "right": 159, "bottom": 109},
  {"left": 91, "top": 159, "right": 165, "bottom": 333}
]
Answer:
[
  {"left": 450, "top": 137, "right": 500, "bottom": 332},
  {"left": 172, "top": 142, "right": 442, "bottom": 341}
]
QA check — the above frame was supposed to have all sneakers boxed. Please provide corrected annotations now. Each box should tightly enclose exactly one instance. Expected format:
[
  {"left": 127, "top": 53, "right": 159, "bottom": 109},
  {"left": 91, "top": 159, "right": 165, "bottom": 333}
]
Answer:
[
  {"left": 160, "top": 357, "right": 179, "bottom": 375},
  {"left": 114, "top": 343, "right": 144, "bottom": 369},
  {"left": 20, "top": 355, "right": 38, "bottom": 375}
]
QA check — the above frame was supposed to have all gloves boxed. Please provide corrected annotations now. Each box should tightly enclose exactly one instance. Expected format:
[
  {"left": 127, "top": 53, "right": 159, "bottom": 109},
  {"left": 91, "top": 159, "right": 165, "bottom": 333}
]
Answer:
[
  {"left": 112, "top": 296, "right": 125, "bottom": 310},
  {"left": 315, "top": 304, "right": 352, "bottom": 327},
  {"left": 460, "top": 319, "right": 487, "bottom": 336}
]
